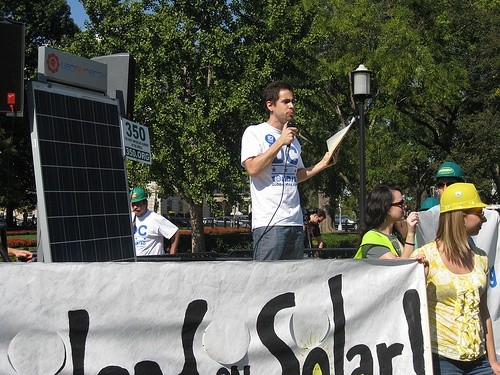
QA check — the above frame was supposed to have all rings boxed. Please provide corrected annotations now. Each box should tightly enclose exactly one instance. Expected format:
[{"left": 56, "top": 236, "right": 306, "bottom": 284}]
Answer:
[{"left": 411, "top": 219, "right": 414, "bottom": 222}]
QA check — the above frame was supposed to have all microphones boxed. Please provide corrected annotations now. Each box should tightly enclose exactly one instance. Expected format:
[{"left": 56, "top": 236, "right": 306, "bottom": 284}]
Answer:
[
  {"left": 287, "top": 121, "right": 295, "bottom": 148},
  {"left": 7, "top": 92, "right": 15, "bottom": 112}
]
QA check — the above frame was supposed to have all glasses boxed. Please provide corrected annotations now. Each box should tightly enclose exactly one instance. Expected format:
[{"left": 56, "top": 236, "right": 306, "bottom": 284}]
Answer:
[
  {"left": 463, "top": 211, "right": 484, "bottom": 218},
  {"left": 391, "top": 199, "right": 404, "bottom": 209},
  {"left": 437, "top": 181, "right": 454, "bottom": 189}
]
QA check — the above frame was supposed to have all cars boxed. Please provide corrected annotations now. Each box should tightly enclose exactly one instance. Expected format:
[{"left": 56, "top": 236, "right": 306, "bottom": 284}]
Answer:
[
  {"left": 166, "top": 215, "right": 192, "bottom": 229},
  {"left": 201, "top": 215, "right": 251, "bottom": 228}
]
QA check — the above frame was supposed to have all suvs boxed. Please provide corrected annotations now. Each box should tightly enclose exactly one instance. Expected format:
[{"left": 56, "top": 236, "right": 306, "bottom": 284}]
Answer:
[{"left": 334, "top": 215, "right": 358, "bottom": 231}]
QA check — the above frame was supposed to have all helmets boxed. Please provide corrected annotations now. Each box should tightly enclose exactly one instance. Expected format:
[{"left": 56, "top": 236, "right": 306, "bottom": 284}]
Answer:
[
  {"left": 130, "top": 187, "right": 147, "bottom": 203},
  {"left": 435, "top": 161, "right": 466, "bottom": 182},
  {"left": 440, "top": 182, "right": 488, "bottom": 213}
]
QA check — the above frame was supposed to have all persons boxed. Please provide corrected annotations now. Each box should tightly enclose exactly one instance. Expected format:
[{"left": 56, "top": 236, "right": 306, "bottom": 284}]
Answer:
[
  {"left": 353, "top": 182, "right": 419, "bottom": 259},
  {"left": 303, "top": 207, "right": 327, "bottom": 257},
  {"left": 410, "top": 183, "right": 500, "bottom": 375},
  {"left": 419, "top": 162, "right": 467, "bottom": 211},
  {"left": 129, "top": 188, "right": 179, "bottom": 256},
  {"left": 241, "top": 80, "right": 340, "bottom": 260},
  {"left": 8, "top": 247, "right": 33, "bottom": 262}
]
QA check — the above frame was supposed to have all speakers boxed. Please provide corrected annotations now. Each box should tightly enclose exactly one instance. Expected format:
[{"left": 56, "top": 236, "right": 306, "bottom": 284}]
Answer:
[
  {"left": 0, "top": 18, "right": 26, "bottom": 120},
  {"left": 90, "top": 53, "right": 136, "bottom": 121}
]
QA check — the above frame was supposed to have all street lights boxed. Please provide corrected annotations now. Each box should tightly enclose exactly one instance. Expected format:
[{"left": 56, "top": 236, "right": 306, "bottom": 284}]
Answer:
[{"left": 350, "top": 64, "right": 373, "bottom": 231}]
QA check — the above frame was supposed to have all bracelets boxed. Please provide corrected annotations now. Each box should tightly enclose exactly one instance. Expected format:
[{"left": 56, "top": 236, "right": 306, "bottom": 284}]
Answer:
[{"left": 405, "top": 242, "right": 415, "bottom": 246}]
